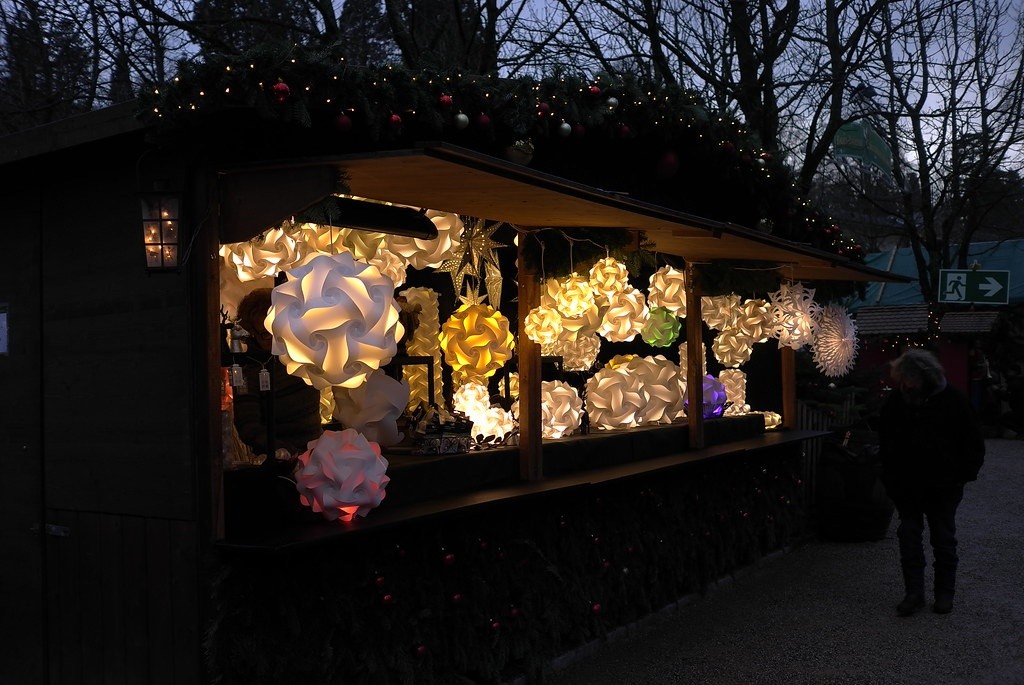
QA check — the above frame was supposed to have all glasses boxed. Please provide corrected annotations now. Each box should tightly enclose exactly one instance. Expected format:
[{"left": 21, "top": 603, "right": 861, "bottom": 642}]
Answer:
[{"left": 248, "top": 322, "right": 274, "bottom": 340}]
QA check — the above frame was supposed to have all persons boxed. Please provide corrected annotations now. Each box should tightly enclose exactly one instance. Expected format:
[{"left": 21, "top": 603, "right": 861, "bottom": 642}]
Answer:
[
  {"left": 881, "top": 350, "right": 988, "bottom": 615},
  {"left": 232, "top": 288, "right": 323, "bottom": 461}
]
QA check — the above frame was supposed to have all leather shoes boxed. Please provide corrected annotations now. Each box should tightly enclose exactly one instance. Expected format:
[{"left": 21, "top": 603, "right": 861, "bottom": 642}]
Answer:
[{"left": 896, "top": 590, "right": 953, "bottom": 614}]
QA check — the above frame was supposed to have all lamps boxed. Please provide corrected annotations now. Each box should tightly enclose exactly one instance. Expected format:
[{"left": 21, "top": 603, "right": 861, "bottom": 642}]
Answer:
[{"left": 142, "top": 159, "right": 864, "bottom": 534}]
